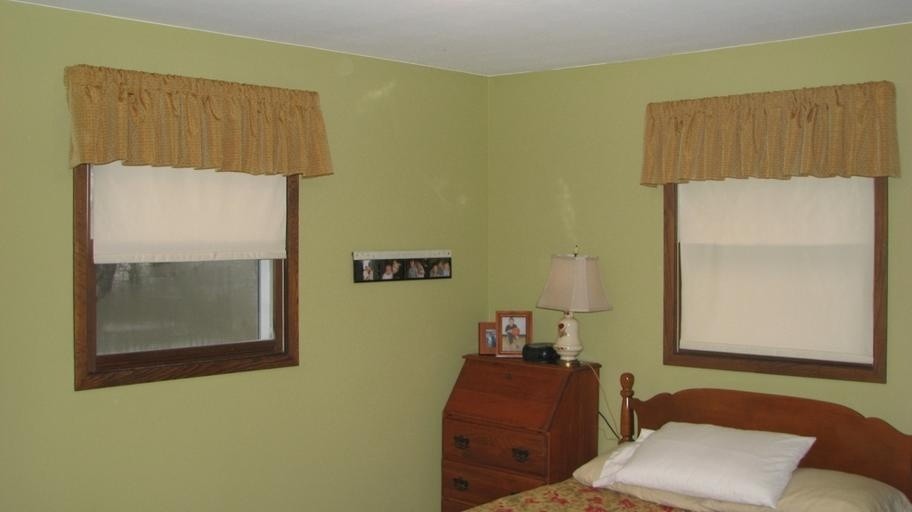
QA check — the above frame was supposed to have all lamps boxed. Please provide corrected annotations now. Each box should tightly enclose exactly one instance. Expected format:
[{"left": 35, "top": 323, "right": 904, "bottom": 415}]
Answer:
[{"left": 535, "top": 246, "right": 614, "bottom": 361}]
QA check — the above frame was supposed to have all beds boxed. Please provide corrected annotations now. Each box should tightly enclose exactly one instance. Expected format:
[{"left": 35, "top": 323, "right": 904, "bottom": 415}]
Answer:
[{"left": 457, "top": 373, "right": 912, "bottom": 511}]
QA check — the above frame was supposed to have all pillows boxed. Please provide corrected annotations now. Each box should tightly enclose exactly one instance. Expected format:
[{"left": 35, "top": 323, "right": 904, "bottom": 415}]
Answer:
[
  {"left": 574, "top": 435, "right": 912, "bottom": 511},
  {"left": 617, "top": 420, "right": 820, "bottom": 511}
]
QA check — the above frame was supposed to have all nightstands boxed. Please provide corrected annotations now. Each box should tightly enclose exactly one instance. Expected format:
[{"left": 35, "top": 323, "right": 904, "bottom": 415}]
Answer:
[{"left": 439, "top": 354, "right": 603, "bottom": 512}]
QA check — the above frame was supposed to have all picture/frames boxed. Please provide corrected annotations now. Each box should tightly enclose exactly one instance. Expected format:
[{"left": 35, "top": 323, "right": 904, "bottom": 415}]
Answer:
[
  {"left": 495, "top": 309, "right": 532, "bottom": 360},
  {"left": 478, "top": 321, "right": 497, "bottom": 356}
]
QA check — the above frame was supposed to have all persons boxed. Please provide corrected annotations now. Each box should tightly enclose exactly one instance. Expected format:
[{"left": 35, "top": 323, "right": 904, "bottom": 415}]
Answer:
[
  {"left": 364, "top": 259, "right": 450, "bottom": 280},
  {"left": 505, "top": 317, "right": 520, "bottom": 348}
]
